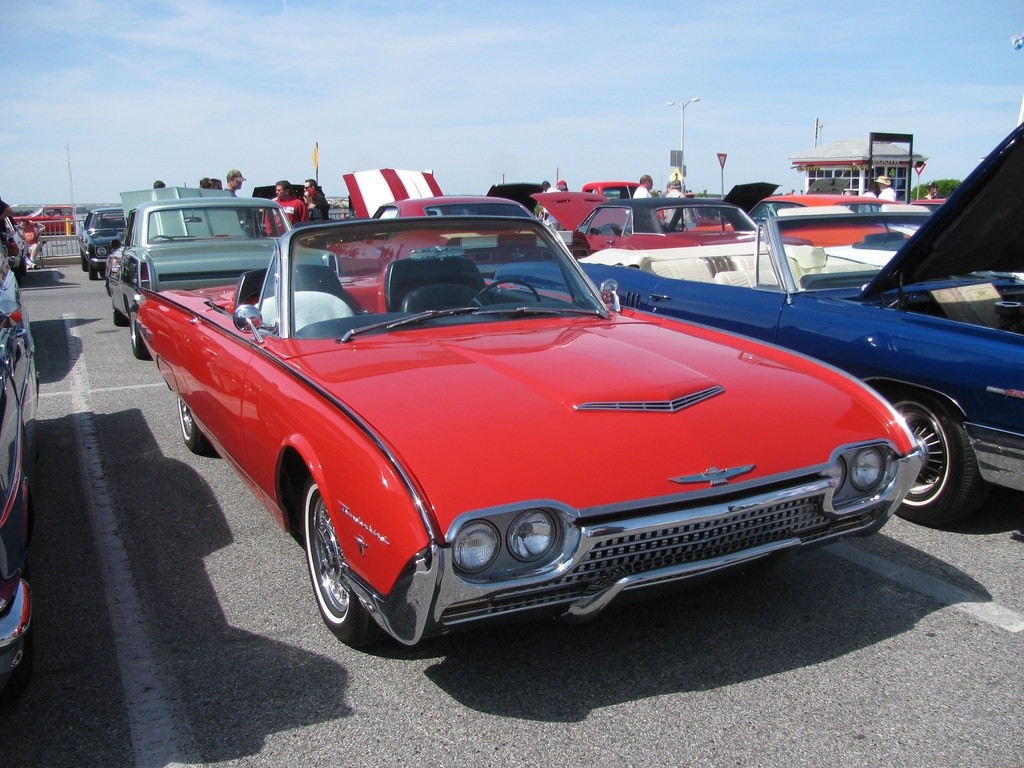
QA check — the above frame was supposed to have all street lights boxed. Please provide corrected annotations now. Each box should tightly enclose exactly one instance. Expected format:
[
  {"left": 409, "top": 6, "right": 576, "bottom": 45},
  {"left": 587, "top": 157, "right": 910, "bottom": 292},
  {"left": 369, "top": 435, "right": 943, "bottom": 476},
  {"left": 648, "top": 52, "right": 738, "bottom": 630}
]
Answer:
[{"left": 666, "top": 97, "right": 701, "bottom": 170}]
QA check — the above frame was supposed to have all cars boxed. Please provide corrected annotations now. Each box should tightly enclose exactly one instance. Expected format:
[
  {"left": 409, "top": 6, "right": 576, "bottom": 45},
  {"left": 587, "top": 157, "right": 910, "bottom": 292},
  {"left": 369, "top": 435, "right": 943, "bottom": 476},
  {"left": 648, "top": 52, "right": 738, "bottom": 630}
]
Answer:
[
  {"left": 326, "top": 199, "right": 349, "bottom": 207},
  {"left": 1, "top": 212, "right": 38, "bottom": 681},
  {"left": 74, "top": 209, "right": 129, "bottom": 279},
  {"left": 531, "top": 182, "right": 946, "bottom": 254},
  {"left": 324, "top": 168, "right": 548, "bottom": 314},
  {"left": 494, "top": 119, "right": 1023, "bottom": 523},
  {"left": 104, "top": 181, "right": 340, "bottom": 359},
  {"left": 14, "top": 205, "right": 77, "bottom": 234},
  {"left": 132, "top": 208, "right": 932, "bottom": 648}
]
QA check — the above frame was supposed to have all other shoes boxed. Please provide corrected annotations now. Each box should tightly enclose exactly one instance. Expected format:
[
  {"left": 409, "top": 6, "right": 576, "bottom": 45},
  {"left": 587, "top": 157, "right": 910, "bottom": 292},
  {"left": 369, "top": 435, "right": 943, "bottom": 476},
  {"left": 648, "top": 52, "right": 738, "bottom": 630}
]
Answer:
[{"left": 27, "top": 263, "right": 37, "bottom": 270}]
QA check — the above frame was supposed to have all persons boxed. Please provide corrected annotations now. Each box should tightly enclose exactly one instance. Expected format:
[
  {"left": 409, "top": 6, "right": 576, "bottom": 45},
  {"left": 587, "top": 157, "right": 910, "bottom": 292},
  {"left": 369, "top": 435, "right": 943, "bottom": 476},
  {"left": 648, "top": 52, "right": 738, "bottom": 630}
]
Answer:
[
  {"left": 265, "top": 180, "right": 310, "bottom": 237},
  {"left": 199, "top": 177, "right": 223, "bottom": 190},
  {"left": 925, "top": 182, "right": 945, "bottom": 199},
  {"left": 225, "top": 170, "right": 247, "bottom": 197},
  {"left": 858, "top": 174, "right": 896, "bottom": 213},
  {"left": 657, "top": 178, "right": 694, "bottom": 227},
  {"left": 541, "top": 179, "right": 569, "bottom": 221},
  {"left": 154, "top": 181, "right": 165, "bottom": 189},
  {"left": 0, "top": 197, "right": 46, "bottom": 270},
  {"left": 302, "top": 179, "right": 330, "bottom": 221},
  {"left": 633, "top": 175, "right": 654, "bottom": 199},
  {"left": 53, "top": 209, "right": 61, "bottom": 217}
]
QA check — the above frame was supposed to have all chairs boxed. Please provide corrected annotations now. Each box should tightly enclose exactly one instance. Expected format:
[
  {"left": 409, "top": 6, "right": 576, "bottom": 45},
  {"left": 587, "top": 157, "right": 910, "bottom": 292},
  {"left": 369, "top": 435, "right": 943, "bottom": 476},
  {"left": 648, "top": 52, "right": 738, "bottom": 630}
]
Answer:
[
  {"left": 715, "top": 269, "right": 777, "bottom": 288},
  {"left": 287, "top": 265, "right": 364, "bottom": 318},
  {"left": 384, "top": 258, "right": 490, "bottom": 314}
]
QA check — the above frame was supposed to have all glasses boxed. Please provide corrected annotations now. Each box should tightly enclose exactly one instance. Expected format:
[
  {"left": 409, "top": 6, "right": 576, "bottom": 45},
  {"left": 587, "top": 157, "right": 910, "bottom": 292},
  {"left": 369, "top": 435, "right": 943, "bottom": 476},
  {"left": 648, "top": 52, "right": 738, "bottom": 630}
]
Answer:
[
  {"left": 304, "top": 195, "right": 311, "bottom": 198},
  {"left": 276, "top": 189, "right": 283, "bottom": 192},
  {"left": 305, "top": 186, "right": 312, "bottom": 190}
]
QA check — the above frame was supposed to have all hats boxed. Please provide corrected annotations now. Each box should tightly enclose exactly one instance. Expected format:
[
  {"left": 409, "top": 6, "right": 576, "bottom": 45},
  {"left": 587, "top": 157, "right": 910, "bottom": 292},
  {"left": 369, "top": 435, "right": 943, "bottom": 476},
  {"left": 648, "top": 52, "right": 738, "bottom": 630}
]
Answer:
[
  {"left": 925, "top": 182, "right": 938, "bottom": 189},
  {"left": 557, "top": 180, "right": 567, "bottom": 189},
  {"left": 228, "top": 170, "right": 246, "bottom": 181},
  {"left": 875, "top": 175, "right": 891, "bottom": 185}
]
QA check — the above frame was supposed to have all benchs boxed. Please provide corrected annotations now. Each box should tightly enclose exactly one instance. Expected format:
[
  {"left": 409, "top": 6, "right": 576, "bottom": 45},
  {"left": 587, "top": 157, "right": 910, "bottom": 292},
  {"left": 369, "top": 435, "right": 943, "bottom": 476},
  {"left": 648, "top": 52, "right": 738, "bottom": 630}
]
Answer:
[{"left": 651, "top": 255, "right": 837, "bottom": 290}]
